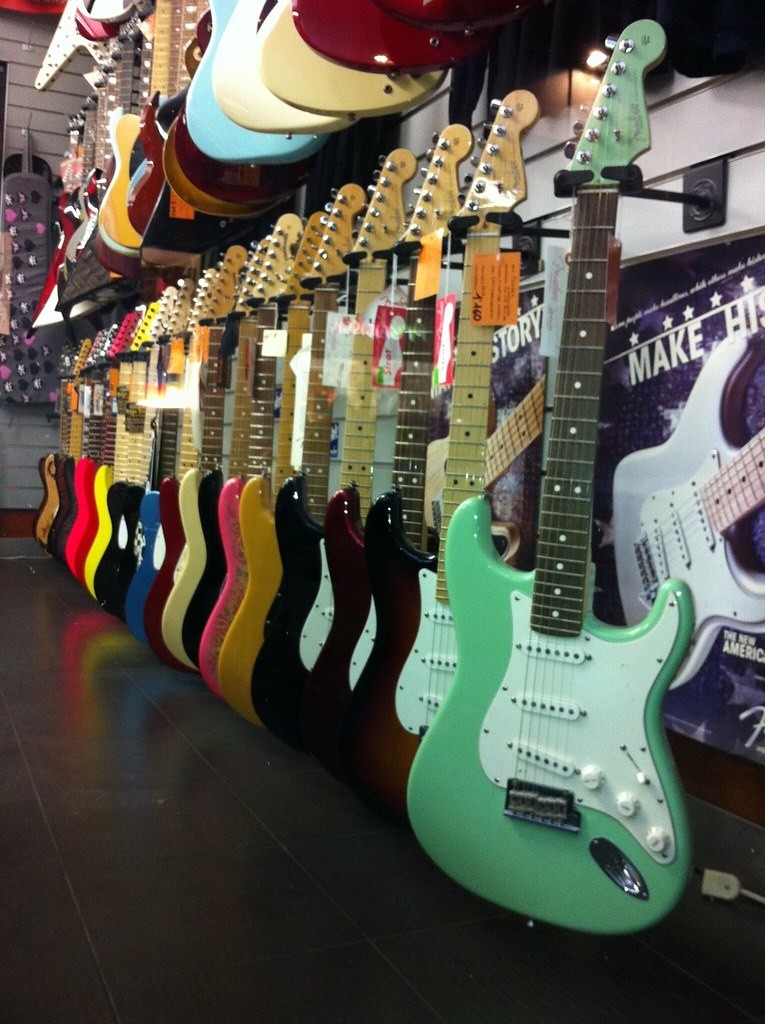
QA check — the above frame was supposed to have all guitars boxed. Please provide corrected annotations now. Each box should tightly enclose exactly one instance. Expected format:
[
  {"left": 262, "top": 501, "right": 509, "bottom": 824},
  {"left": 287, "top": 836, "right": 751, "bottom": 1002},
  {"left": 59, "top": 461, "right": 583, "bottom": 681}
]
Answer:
[
  {"left": 33, "top": 0, "right": 541, "bottom": 838},
  {"left": 404, "top": 18, "right": 695, "bottom": 938}
]
